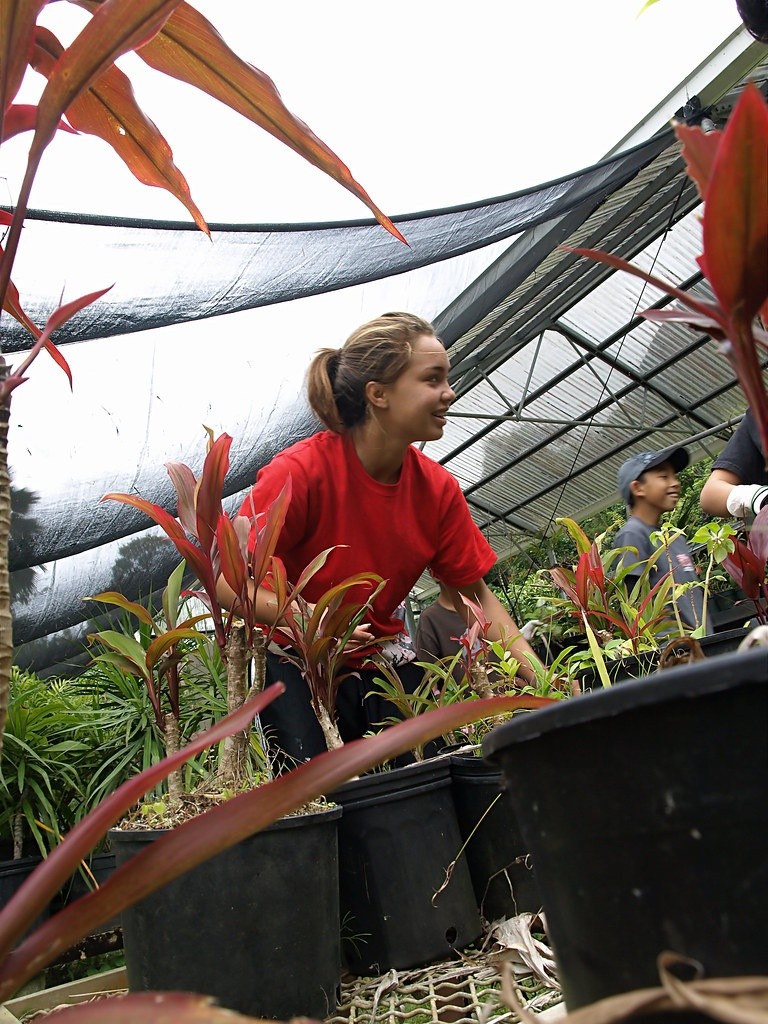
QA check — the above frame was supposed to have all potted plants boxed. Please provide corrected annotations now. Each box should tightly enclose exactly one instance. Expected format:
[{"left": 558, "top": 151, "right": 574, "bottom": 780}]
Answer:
[
  {"left": 54, "top": 680, "right": 141, "bottom": 932},
  {"left": 0, "top": 668, "right": 83, "bottom": 948},
  {"left": 438, "top": 590, "right": 579, "bottom": 930},
  {"left": 81, "top": 424, "right": 350, "bottom": 1021},
  {"left": 535, "top": 518, "right": 754, "bottom": 692},
  {"left": 236, "top": 563, "right": 483, "bottom": 975}
]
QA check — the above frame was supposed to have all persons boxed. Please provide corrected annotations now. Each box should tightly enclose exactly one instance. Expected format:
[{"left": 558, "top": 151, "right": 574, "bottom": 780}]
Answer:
[
  {"left": 202, "top": 308, "right": 585, "bottom": 783},
  {"left": 699, "top": 401, "right": 768, "bottom": 515},
  {"left": 613, "top": 444, "right": 714, "bottom": 644}
]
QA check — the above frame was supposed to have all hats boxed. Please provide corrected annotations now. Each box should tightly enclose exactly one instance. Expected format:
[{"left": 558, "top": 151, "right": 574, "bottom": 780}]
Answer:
[{"left": 617, "top": 446, "right": 690, "bottom": 504}]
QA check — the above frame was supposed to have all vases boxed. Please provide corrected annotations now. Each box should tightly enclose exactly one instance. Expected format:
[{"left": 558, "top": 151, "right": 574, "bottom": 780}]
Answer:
[
  {"left": 326, "top": 768, "right": 483, "bottom": 976},
  {"left": 518, "top": 570, "right": 595, "bottom": 673},
  {"left": 0, "top": 866, "right": 50, "bottom": 949},
  {"left": 693, "top": 570, "right": 768, "bottom": 613},
  {"left": 62, "top": 865, "right": 120, "bottom": 935},
  {"left": 449, "top": 767, "right": 545, "bottom": 921},
  {"left": 481, "top": 647, "right": 768, "bottom": 1024}
]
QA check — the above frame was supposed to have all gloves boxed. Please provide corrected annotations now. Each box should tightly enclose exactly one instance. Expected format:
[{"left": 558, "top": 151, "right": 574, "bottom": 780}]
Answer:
[{"left": 727, "top": 484, "right": 768, "bottom": 517}]
